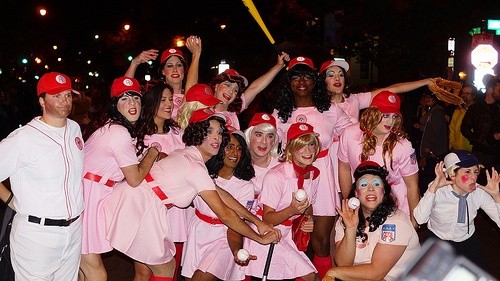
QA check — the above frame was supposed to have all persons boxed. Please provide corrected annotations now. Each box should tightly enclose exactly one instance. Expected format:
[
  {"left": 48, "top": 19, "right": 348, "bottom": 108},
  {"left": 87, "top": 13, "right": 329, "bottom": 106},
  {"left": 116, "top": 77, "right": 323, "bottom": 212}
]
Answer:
[
  {"left": 0, "top": 35, "right": 500, "bottom": 281},
  {"left": 318, "top": 160, "right": 422, "bottom": 281},
  {"left": 1, "top": 71, "right": 85, "bottom": 281},
  {"left": 105, "top": 108, "right": 283, "bottom": 281}
]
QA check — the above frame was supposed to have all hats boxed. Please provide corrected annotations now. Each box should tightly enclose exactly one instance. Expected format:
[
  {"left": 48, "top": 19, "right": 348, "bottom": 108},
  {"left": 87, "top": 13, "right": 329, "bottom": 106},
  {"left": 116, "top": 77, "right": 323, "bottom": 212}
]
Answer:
[
  {"left": 444, "top": 151, "right": 485, "bottom": 169},
  {"left": 289, "top": 57, "right": 314, "bottom": 70},
  {"left": 111, "top": 77, "right": 142, "bottom": 97},
  {"left": 226, "top": 69, "right": 248, "bottom": 87},
  {"left": 189, "top": 108, "right": 226, "bottom": 124},
  {"left": 160, "top": 49, "right": 184, "bottom": 63},
  {"left": 186, "top": 83, "right": 223, "bottom": 106},
  {"left": 37, "top": 72, "right": 80, "bottom": 96},
  {"left": 370, "top": 91, "right": 401, "bottom": 114},
  {"left": 248, "top": 113, "right": 276, "bottom": 128},
  {"left": 287, "top": 123, "right": 319, "bottom": 143},
  {"left": 320, "top": 60, "right": 349, "bottom": 74},
  {"left": 226, "top": 125, "right": 247, "bottom": 140}
]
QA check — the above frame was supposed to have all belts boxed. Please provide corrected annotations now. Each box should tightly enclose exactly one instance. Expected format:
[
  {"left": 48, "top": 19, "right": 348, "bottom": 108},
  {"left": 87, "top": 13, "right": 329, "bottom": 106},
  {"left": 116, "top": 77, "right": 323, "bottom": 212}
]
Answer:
[
  {"left": 256, "top": 208, "right": 293, "bottom": 227},
  {"left": 317, "top": 149, "right": 328, "bottom": 158},
  {"left": 333, "top": 136, "right": 340, "bottom": 142},
  {"left": 195, "top": 208, "right": 223, "bottom": 224},
  {"left": 83, "top": 172, "right": 115, "bottom": 187},
  {"left": 28, "top": 215, "right": 79, "bottom": 227},
  {"left": 145, "top": 173, "right": 173, "bottom": 209}
]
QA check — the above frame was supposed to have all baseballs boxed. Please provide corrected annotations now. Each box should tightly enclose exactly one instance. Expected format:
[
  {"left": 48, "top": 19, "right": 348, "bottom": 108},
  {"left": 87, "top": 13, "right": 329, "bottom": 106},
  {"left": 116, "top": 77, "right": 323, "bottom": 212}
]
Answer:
[
  {"left": 192, "top": 36, "right": 198, "bottom": 45},
  {"left": 237, "top": 249, "right": 249, "bottom": 262},
  {"left": 295, "top": 189, "right": 308, "bottom": 202},
  {"left": 347, "top": 196, "right": 360, "bottom": 210}
]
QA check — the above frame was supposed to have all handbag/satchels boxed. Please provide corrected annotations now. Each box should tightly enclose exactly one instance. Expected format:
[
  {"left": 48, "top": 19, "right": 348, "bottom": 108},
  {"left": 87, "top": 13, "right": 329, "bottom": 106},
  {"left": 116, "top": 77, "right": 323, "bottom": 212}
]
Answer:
[{"left": 292, "top": 216, "right": 310, "bottom": 251}]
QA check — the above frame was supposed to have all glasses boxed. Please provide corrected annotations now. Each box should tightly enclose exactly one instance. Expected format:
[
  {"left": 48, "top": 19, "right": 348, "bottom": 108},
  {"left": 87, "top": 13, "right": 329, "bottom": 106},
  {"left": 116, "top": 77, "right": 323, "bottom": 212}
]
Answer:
[{"left": 145, "top": 79, "right": 167, "bottom": 92}]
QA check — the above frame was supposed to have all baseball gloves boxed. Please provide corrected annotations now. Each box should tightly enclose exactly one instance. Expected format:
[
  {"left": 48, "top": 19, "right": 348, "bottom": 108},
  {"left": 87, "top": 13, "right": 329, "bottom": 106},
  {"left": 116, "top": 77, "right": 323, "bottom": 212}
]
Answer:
[{"left": 428, "top": 78, "right": 463, "bottom": 106}]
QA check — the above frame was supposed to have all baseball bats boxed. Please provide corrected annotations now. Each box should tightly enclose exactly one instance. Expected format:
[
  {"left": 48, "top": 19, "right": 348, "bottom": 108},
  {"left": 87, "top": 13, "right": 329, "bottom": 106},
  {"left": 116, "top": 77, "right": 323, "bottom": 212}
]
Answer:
[
  {"left": 242, "top": 0, "right": 289, "bottom": 67},
  {"left": 261, "top": 243, "right": 276, "bottom": 281}
]
